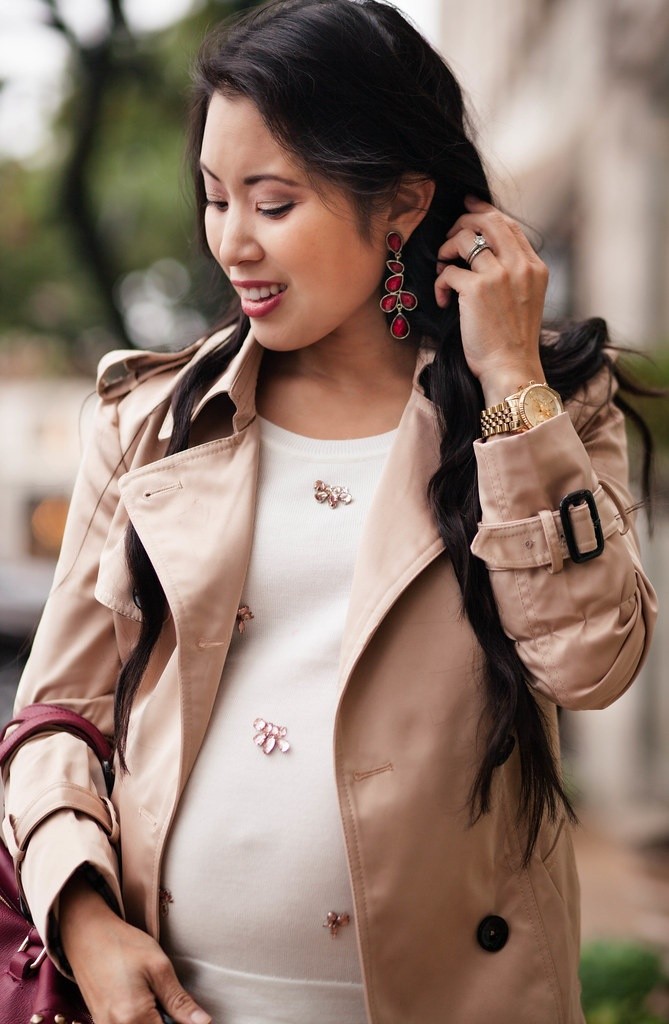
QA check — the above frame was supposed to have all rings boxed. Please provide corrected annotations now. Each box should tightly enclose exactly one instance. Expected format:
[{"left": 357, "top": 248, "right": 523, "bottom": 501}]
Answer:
[{"left": 466, "top": 235, "right": 491, "bottom": 266}]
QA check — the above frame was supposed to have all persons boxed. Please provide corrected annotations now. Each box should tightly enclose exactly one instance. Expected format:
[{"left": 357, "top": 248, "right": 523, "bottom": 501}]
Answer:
[{"left": 0, "top": 0, "right": 658, "bottom": 1024}]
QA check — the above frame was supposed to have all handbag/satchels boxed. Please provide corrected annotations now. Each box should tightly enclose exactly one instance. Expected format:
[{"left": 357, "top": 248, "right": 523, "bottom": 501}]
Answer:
[{"left": 0, "top": 703, "right": 112, "bottom": 1024}]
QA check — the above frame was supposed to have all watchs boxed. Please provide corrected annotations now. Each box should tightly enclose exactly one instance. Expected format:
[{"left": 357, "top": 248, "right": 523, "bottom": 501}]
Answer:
[{"left": 480, "top": 379, "right": 564, "bottom": 441}]
